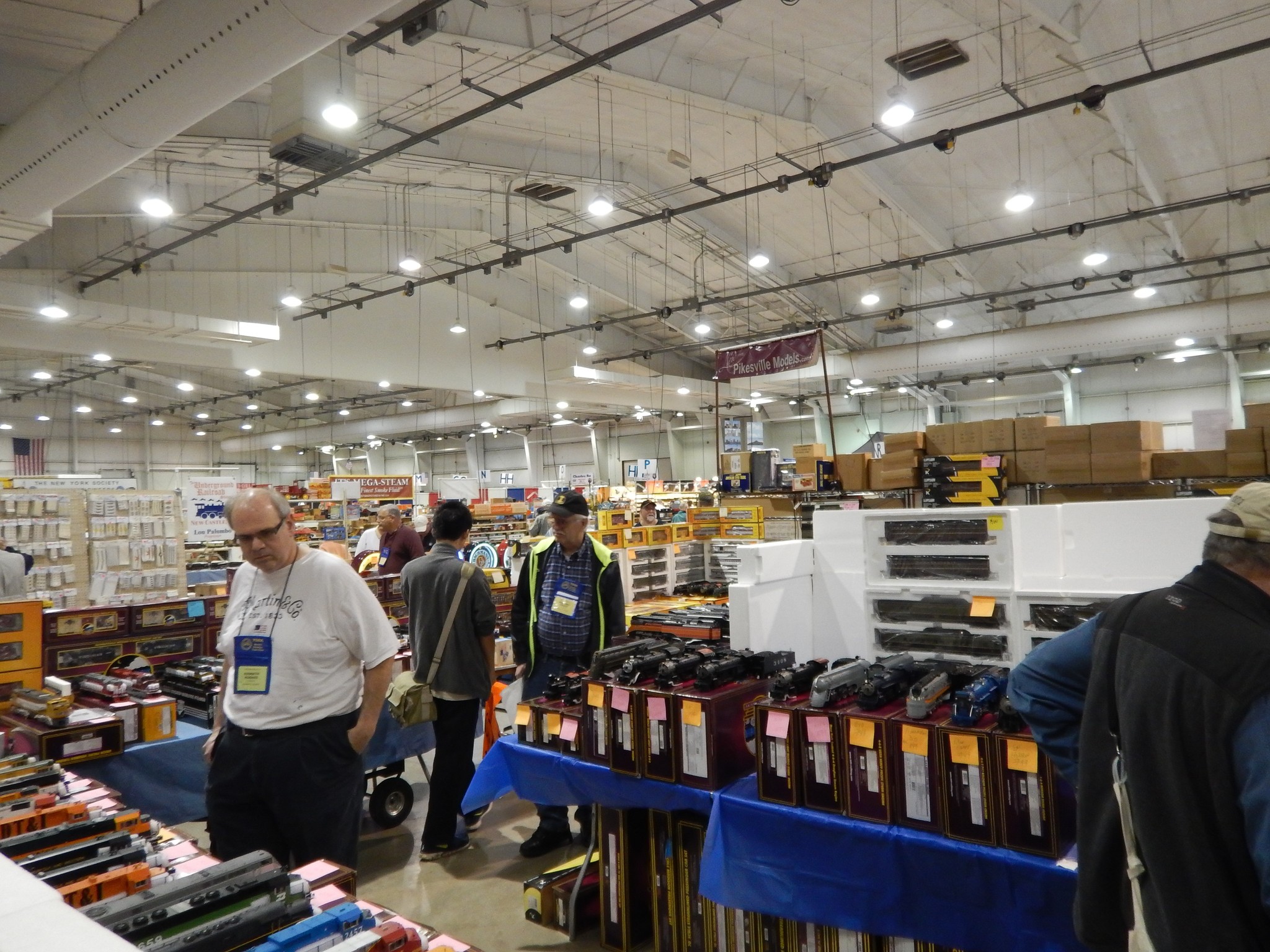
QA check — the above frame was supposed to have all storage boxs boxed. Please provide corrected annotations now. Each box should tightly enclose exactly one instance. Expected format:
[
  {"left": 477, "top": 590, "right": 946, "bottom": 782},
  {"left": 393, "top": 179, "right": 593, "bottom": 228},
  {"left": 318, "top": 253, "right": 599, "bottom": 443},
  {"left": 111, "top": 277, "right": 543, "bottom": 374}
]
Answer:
[{"left": 0, "top": 394, "right": 1270, "bottom": 952}]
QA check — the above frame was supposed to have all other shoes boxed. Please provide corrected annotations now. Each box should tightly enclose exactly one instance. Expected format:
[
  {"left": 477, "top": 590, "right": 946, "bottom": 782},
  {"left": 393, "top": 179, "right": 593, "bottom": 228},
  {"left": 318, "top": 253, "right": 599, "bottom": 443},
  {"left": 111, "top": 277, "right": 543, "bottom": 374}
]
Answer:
[
  {"left": 518, "top": 821, "right": 573, "bottom": 857},
  {"left": 576, "top": 825, "right": 598, "bottom": 847}
]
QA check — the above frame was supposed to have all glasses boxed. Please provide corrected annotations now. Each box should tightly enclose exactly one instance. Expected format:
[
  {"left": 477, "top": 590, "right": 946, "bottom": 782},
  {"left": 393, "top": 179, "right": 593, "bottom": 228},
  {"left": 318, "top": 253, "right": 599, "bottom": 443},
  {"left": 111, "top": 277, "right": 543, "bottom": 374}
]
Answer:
[
  {"left": 551, "top": 516, "right": 580, "bottom": 527},
  {"left": 232, "top": 513, "right": 286, "bottom": 547},
  {"left": 376, "top": 514, "right": 394, "bottom": 522},
  {"left": 641, "top": 507, "right": 655, "bottom": 512}
]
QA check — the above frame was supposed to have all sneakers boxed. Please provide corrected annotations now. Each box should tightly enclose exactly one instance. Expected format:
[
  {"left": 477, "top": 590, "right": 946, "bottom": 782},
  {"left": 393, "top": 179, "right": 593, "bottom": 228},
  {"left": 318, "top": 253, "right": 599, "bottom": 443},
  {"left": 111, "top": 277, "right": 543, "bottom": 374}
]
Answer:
[
  {"left": 418, "top": 830, "right": 470, "bottom": 859},
  {"left": 464, "top": 799, "right": 493, "bottom": 830}
]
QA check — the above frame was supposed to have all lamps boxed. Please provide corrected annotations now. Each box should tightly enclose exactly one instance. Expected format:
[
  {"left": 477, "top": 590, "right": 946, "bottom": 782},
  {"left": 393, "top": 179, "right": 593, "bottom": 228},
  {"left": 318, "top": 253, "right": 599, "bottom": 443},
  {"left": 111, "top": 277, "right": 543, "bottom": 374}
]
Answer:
[
  {"left": 567, "top": 187, "right": 590, "bottom": 310},
  {"left": 447, "top": 230, "right": 468, "bottom": 336},
  {"left": 398, "top": 166, "right": 422, "bottom": 274},
  {"left": 1132, "top": 237, "right": 1158, "bottom": 300},
  {"left": 582, "top": 282, "right": 598, "bottom": 356},
  {"left": 932, "top": 277, "right": 955, "bottom": 331},
  {"left": 857, "top": 213, "right": 881, "bottom": 307},
  {"left": 316, "top": 38, "right": 360, "bottom": 131},
  {"left": 878, "top": 0, "right": 916, "bottom": 131},
  {"left": 746, "top": 115, "right": 770, "bottom": 270},
  {"left": 587, "top": 75, "right": 614, "bottom": 220},
  {"left": 1003, "top": 25, "right": 1035, "bottom": 214},
  {"left": 37, "top": 227, "right": 69, "bottom": 322},
  {"left": 280, "top": 221, "right": 303, "bottom": 309},
  {"left": 1080, "top": 155, "right": 1109, "bottom": 268},
  {"left": 135, "top": 149, "right": 175, "bottom": 220}
]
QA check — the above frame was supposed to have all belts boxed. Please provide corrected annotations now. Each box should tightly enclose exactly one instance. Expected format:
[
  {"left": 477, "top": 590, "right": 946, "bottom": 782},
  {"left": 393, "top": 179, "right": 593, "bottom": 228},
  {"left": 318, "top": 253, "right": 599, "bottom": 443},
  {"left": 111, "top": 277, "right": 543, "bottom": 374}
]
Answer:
[{"left": 227, "top": 715, "right": 350, "bottom": 737}]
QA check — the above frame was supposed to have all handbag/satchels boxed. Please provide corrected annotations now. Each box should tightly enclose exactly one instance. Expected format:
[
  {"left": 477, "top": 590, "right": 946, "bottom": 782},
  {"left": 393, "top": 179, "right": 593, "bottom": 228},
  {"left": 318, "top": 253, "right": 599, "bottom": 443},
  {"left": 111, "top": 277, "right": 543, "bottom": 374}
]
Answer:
[
  {"left": 384, "top": 670, "right": 438, "bottom": 729},
  {"left": 499, "top": 675, "right": 524, "bottom": 734},
  {"left": 482, "top": 682, "right": 508, "bottom": 759}
]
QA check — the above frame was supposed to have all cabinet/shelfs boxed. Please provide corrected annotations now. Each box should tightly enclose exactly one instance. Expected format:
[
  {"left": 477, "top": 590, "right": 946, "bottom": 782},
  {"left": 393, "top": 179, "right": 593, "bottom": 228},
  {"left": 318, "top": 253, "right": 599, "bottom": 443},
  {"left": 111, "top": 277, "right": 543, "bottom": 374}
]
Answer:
[{"left": 466, "top": 515, "right": 529, "bottom": 544}]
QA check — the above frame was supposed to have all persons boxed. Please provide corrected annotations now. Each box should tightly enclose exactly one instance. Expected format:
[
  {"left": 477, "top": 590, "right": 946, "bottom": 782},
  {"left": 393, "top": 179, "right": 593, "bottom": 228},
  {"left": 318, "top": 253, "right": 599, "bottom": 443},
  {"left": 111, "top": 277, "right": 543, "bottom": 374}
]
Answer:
[
  {"left": 1005, "top": 482, "right": 1270, "bottom": 952},
  {"left": 0, "top": 540, "right": 35, "bottom": 601},
  {"left": 354, "top": 503, "right": 436, "bottom": 577},
  {"left": 400, "top": 501, "right": 497, "bottom": 861},
  {"left": 528, "top": 491, "right": 715, "bottom": 537},
  {"left": 510, "top": 490, "right": 626, "bottom": 856},
  {"left": 202, "top": 487, "right": 402, "bottom": 869}
]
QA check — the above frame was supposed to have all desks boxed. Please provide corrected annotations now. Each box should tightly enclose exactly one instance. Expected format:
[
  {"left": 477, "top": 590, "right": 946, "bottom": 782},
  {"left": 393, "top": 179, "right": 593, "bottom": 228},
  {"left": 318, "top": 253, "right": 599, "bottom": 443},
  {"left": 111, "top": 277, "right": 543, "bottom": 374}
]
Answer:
[{"left": 450, "top": 739, "right": 1090, "bottom": 952}]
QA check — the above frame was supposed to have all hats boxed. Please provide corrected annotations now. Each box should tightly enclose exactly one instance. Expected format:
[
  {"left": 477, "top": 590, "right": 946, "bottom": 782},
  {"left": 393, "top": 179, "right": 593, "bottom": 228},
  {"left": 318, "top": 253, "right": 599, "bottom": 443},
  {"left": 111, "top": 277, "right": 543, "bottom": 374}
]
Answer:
[
  {"left": 697, "top": 491, "right": 714, "bottom": 501},
  {"left": 640, "top": 500, "right": 656, "bottom": 508},
  {"left": 537, "top": 507, "right": 544, "bottom": 514},
  {"left": 414, "top": 515, "right": 428, "bottom": 532},
  {"left": 542, "top": 490, "right": 589, "bottom": 518},
  {"left": 1208, "top": 482, "right": 1270, "bottom": 542},
  {"left": 542, "top": 499, "right": 552, "bottom": 507}
]
